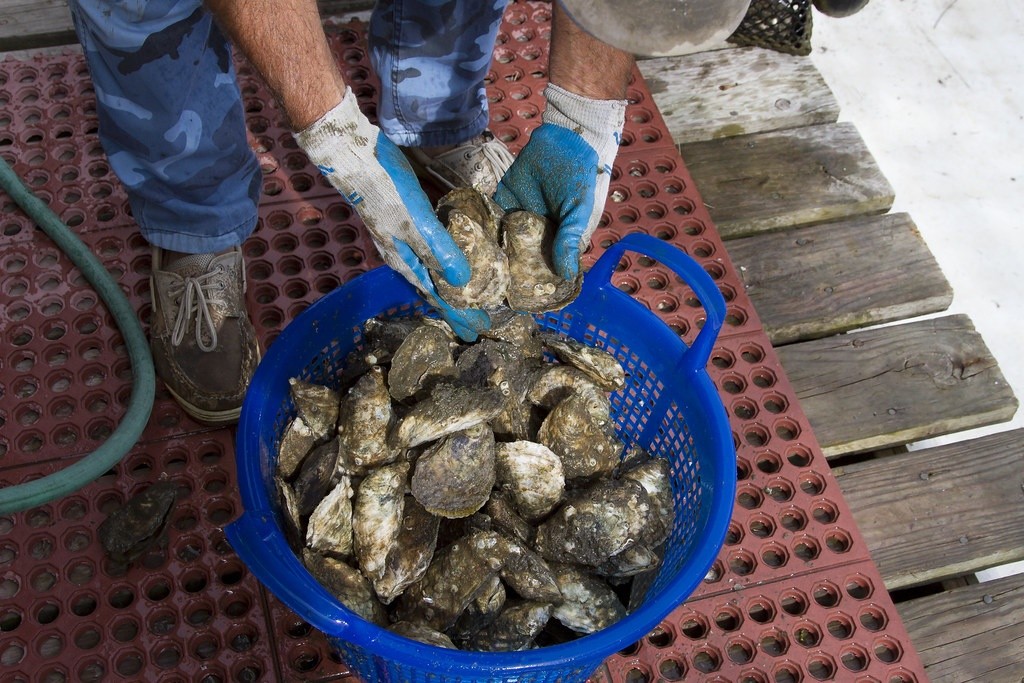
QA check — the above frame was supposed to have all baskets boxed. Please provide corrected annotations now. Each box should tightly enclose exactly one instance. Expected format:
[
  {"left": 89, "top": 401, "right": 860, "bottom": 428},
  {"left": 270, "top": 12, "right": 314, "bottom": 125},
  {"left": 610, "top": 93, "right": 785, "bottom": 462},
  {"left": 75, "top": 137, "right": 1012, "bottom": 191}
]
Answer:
[{"left": 224, "top": 230, "right": 741, "bottom": 682}]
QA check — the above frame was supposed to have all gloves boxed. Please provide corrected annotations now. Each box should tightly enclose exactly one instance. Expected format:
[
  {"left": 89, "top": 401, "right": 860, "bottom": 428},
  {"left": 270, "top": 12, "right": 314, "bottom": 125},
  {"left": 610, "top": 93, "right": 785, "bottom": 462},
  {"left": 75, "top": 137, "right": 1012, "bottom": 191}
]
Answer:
[
  {"left": 492, "top": 82, "right": 628, "bottom": 281},
  {"left": 292, "top": 84, "right": 492, "bottom": 343}
]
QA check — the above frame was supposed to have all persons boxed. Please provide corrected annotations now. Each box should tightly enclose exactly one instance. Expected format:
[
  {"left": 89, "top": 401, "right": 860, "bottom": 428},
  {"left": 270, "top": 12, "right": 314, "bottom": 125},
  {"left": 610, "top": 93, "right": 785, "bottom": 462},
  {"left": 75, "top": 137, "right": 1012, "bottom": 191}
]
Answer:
[{"left": 65, "top": 0, "right": 753, "bottom": 422}]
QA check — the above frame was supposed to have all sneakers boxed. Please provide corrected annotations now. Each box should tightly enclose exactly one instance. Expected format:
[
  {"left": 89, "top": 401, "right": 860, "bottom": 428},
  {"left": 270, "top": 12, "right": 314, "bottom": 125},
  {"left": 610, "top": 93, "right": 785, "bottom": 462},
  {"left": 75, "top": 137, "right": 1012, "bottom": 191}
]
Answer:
[
  {"left": 405, "top": 127, "right": 514, "bottom": 205},
  {"left": 148, "top": 241, "right": 262, "bottom": 421}
]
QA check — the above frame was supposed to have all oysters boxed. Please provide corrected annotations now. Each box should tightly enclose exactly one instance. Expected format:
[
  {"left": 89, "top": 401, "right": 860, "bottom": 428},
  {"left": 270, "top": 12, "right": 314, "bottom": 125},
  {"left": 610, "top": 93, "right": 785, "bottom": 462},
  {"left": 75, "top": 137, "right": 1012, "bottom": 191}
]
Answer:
[
  {"left": 277, "top": 308, "right": 676, "bottom": 654},
  {"left": 430, "top": 188, "right": 584, "bottom": 313}
]
